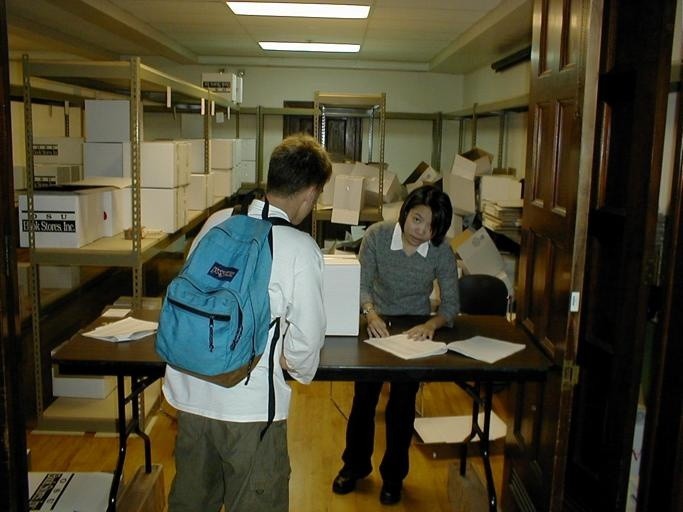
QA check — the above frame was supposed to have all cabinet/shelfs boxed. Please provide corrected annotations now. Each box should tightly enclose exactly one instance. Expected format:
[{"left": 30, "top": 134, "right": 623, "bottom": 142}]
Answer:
[
  {"left": 312, "top": 88, "right": 387, "bottom": 241},
  {"left": 441, "top": 94, "right": 530, "bottom": 243},
  {"left": 239, "top": 105, "right": 441, "bottom": 243},
  {"left": 22, "top": 57, "right": 240, "bottom": 432}
]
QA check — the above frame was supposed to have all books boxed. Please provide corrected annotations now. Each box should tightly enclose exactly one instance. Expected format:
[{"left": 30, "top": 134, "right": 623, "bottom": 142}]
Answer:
[
  {"left": 445, "top": 336, "right": 525, "bottom": 365},
  {"left": 480, "top": 200, "right": 523, "bottom": 233},
  {"left": 363, "top": 333, "right": 448, "bottom": 360}
]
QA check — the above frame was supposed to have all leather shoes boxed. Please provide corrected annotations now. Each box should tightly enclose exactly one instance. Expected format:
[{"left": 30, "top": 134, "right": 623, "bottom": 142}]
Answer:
[
  {"left": 379, "top": 476, "right": 404, "bottom": 506},
  {"left": 331, "top": 463, "right": 374, "bottom": 494}
]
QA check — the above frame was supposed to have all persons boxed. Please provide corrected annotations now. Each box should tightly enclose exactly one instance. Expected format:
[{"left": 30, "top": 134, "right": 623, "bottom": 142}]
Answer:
[
  {"left": 333, "top": 186, "right": 461, "bottom": 504},
  {"left": 165, "top": 130, "right": 332, "bottom": 512}
]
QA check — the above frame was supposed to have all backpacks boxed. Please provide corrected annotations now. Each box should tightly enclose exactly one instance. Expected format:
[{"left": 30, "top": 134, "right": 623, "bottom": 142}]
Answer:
[{"left": 152, "top": 193, "right": 300, "bottom": 388}]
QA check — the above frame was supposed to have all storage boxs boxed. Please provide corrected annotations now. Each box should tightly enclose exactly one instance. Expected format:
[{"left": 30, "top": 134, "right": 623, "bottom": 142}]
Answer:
[
  {"left": 19, "top": 98, "right": 255, "bottom": 247},
  {"left": 318, "top": 148, "right": 524, "bottom": 297}
]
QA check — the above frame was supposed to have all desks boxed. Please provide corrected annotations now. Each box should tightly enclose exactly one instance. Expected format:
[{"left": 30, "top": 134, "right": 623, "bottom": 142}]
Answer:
[{"left": 51, "top": 306, "right": 555, "bottom": 512}]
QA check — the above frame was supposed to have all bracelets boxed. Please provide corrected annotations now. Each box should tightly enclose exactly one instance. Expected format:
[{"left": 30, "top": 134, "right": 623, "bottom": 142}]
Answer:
[{"left": 361, "top": 306, "right": 375, "bottom": 318}]
